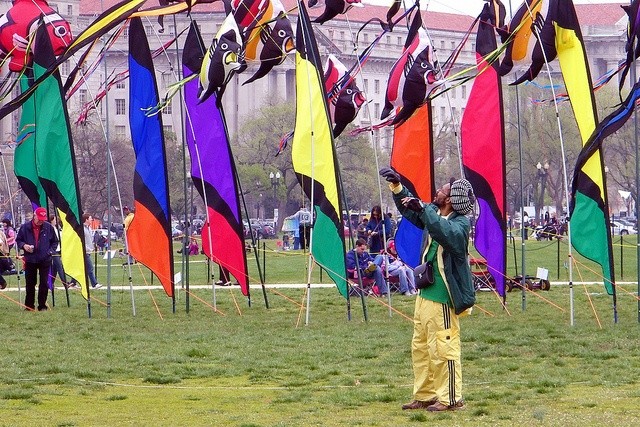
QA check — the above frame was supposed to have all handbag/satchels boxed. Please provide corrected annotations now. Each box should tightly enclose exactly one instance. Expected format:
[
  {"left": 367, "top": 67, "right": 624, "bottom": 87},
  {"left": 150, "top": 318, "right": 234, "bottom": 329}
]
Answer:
[
  {"left": 367, "top": 220, "right": 382, "bottom": 249},
  {"left": 411, "top": 262, "right": 435, "bottom": 289}
]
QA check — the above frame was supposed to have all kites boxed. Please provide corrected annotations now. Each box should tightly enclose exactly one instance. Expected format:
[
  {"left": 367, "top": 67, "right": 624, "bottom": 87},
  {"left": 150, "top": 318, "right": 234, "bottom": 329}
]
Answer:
[
  {"left": 233, "top": 1, "right": 298, "bottom": 86},
  {"left": 141, "top": 12, "right": 244, "bottom": 119},
  {"left": 346, "top": 14, "right": 445, "bottom": 136},
  {"left": 270, "top": 51, "right": 368, "bottom": 157},
  {"left": 304, "top": 1, "right": 367, "bottom": 27},
  {"left": 528, "top": 3, "right": 640, "bottom": 110},
  {"left": 0, "top": 1, "right": 74, "bottom": 100}
]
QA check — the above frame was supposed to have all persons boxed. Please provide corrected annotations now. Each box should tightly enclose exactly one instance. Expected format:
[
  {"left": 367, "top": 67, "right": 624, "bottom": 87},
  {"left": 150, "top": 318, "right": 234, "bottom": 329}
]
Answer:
[
  {"left": 357, "top": 218, "right": 368, "bottom": 238},
  {"left": 83, "top": 213, "right": 103, "bottom": 288},
  {"left": 378, "top": 167, "right": 477, "bottom": 411},
  {"left": 366, "top": 205, "right": 391, "bottom": 257},
  {"left": 387, "top": 212, "right": 397, "bottom": 235},
  {"left": 524, "top": 211, "right": 529, "bottom": 239},
  {"left": 345, "top": 236, "right": 389, "bottom": 297},
  {"left": 287, "top": 205, "right": 312, "bottom": 250},
  {"left": 98, "top": 235, "right": 104, "bottom": 255},
  {"left": 559, "top": 212, "right": 570, "bottom": 235},
  {"left": 15, "top": 207, "right": 79, "bottom": 311},
  {"left": 382, "top": 239, "right": 420, "bottom": 298},
  {"left": 122, "top": 205, "right": 134, "bottom": 265},
  {"left": 215, "top": 264, "right": 231, "bottom": 287},
  {"left": 2, "top": 217, "right": 15, "bottom": 273},
  {"left": 0, "top": 223, "right": 9, "bottom": 289},
  {"left": 105, "top": 234, "right": 110, "bottom": 250}
]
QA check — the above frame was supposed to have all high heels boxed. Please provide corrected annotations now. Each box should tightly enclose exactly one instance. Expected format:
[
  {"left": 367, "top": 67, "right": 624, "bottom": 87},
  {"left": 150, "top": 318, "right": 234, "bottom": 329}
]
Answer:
[
  {"left": 223, "top": 281, "right": 231, "bottom": 286},
  {"left": 215, "top": 280, "right": 223, "bottom": 285}
]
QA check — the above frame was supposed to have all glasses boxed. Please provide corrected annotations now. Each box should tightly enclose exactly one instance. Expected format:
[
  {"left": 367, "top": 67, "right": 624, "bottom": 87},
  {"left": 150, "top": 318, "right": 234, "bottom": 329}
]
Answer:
[{"left": 439, "top": 188, "right": 448, "bottom": 196}]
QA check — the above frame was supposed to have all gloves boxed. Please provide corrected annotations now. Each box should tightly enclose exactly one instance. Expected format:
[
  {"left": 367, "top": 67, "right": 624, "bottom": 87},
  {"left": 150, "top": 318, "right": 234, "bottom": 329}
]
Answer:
[{"left": 380, "top": 166, "right": 401, "bottom": 185}]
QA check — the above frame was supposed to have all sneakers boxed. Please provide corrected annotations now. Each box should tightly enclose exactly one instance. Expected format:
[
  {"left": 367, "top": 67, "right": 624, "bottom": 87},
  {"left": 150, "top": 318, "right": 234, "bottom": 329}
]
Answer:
[
  {"left": 232, "top": 281, "right": 238, "bottom": 285},
  {"left": 92, "top": 283, "right": 103, "bottom": 290},
  {"left": 364, "top": 263, "right": 376, "bottom": 273},
  {"left": 402, "top": 398, "right": 437, "bottom": 409},
  {"left": 427, "top": 399, "right": 464, "bottom": 411},
  {"left": 403, "top": 290, "right": 412, "bottom": 296},
  {"left": 411, "top": 290, "right": 417, "bottom": 294}
]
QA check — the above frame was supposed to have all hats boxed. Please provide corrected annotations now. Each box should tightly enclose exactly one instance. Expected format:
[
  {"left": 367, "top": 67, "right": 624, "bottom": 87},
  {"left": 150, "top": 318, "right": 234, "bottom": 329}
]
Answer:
[
  {"left": 34, "top": 207, "right": 47, "bottom": 221},
  {"left": 449, "top": 176, "right": 476, "bottom": 217}
]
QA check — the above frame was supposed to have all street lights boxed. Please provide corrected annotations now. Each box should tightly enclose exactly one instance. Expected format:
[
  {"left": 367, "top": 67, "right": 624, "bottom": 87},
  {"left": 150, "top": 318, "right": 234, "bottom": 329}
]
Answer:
[
  {"left": 187, "top": 172, "right": 197, "bottom": 231},
  {"left": 270, "top": 171, "right": 281, "bottom": 223},
  {"left": 536, "top": 161, "right": 550, "bottom": 227}
]
